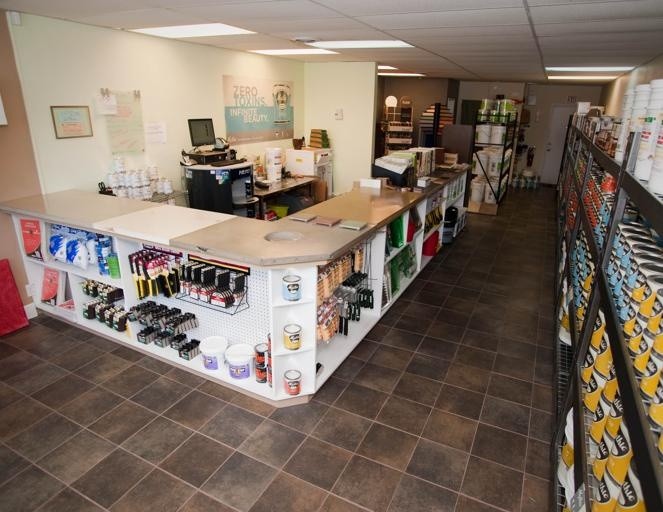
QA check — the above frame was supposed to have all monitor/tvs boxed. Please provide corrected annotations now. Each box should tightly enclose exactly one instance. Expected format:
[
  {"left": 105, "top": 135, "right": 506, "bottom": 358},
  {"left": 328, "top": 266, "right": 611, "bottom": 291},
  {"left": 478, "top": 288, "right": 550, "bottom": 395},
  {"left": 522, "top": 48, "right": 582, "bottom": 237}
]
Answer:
[{"left": 187, "top": 117, "right": 216, "bottom": 153}]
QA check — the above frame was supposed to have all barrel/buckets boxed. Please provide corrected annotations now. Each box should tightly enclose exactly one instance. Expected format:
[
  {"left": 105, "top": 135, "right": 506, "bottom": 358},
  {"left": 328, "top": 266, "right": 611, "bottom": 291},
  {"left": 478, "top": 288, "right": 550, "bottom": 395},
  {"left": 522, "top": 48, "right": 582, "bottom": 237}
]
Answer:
[
  {"left": 470, "top": 123, "right": 509, "bottom": 204},
  {"left": 614, "top": 78, "right": 663, "bottom": 196},
  {"left": 264, "top": 147, "right": 283, "bottom": 166},
  {"left": 225, "top": 343, "right": 255, "bottom": 380},
  {"left": 266, "top": 203, "right": 290, "bottom": 219},
  {"left": 199, "top": 336, "right": 228, "bottom": 371},
  {"left": 265, "top": 165, "right": 283, "bottom": 183}
]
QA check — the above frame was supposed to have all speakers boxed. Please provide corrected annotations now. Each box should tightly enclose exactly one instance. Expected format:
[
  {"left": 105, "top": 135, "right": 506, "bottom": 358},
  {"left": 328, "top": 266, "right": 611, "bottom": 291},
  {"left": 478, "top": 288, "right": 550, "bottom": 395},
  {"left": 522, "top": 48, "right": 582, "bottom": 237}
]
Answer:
[{"left": 226, "top": 149, "right": 236, "bottom": 160}]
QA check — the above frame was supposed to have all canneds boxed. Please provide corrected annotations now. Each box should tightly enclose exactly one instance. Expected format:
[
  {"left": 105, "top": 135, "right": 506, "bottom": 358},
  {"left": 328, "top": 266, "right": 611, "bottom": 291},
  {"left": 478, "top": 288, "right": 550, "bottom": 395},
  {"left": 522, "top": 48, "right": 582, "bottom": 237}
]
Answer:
[
  {"left": 254, "top": 332, "right": 272, "bottom": 387},
  {"left": 282, "top": 275, "right": 302, "bottom": 300},
  {"left": 283, "top": 323, "right": 302, "bottom": 350}
]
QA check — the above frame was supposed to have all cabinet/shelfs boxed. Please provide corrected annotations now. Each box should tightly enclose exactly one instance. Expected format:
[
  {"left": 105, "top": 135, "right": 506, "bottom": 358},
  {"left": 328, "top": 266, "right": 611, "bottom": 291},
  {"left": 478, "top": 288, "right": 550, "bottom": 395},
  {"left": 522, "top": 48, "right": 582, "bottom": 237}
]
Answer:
[{"left": 381, "top": 90, "right": 527, "bottom": 218}]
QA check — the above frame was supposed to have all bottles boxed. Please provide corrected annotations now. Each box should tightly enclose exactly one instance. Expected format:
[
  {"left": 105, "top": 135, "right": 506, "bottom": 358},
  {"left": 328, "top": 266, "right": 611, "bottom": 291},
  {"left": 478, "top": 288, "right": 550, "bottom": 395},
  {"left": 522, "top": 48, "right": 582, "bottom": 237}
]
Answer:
[
  {"left": 254, "top": 155, "right": 263, "bottom": 177},
  {"left": 105, "top": 151, "right": 174, "bottom": 202}
]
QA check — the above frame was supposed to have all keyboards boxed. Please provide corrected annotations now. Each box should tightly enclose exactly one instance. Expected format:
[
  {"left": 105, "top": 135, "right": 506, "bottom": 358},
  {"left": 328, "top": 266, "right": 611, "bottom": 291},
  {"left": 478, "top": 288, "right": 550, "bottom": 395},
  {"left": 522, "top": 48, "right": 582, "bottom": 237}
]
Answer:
[{"left": 210, "top": 159, "right": 244, "bottom": 167}]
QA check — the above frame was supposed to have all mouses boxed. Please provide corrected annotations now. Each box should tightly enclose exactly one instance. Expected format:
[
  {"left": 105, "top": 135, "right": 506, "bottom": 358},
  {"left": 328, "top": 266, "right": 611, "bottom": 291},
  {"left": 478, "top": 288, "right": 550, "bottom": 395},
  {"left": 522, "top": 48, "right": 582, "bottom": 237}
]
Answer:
[{"left": 240, "top": 158, "right": 247, "bottom": 162}]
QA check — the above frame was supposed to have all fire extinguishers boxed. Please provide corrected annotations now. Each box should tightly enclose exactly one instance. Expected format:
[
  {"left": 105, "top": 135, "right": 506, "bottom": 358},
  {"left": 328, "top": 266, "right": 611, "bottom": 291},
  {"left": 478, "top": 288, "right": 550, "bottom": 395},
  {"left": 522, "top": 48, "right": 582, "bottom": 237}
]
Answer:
[{"left": 527, "top": 146, "right": 536, "bottom": 167}]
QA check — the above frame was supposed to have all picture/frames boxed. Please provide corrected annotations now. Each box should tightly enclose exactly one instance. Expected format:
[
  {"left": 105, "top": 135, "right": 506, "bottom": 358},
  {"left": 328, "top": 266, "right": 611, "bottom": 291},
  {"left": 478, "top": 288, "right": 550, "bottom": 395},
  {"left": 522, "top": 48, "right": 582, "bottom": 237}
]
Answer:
[{"left": 48, "top": 104, "right": 95, "bottom": 141}]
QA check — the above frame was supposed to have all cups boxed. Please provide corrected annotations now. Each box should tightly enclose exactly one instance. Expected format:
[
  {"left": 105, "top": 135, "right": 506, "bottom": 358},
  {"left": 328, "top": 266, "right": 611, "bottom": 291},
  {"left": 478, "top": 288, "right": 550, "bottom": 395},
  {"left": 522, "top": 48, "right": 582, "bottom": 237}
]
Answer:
[
  {"left": 282, "top": 323, "right": 303, "bottom": 352},
  {"left": 292, "top": 138, "right": 303, "bottom": 151},
  {"left": 280, "top": 272, "right": 302, "bottom": 303}
]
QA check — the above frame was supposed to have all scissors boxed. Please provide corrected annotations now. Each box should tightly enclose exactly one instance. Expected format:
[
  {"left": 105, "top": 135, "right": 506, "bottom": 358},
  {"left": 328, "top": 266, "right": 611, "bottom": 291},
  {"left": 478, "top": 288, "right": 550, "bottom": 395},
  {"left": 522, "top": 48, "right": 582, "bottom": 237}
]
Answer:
[{"left": 98, "top": 181, "right": 105, "bottom": 191}]
